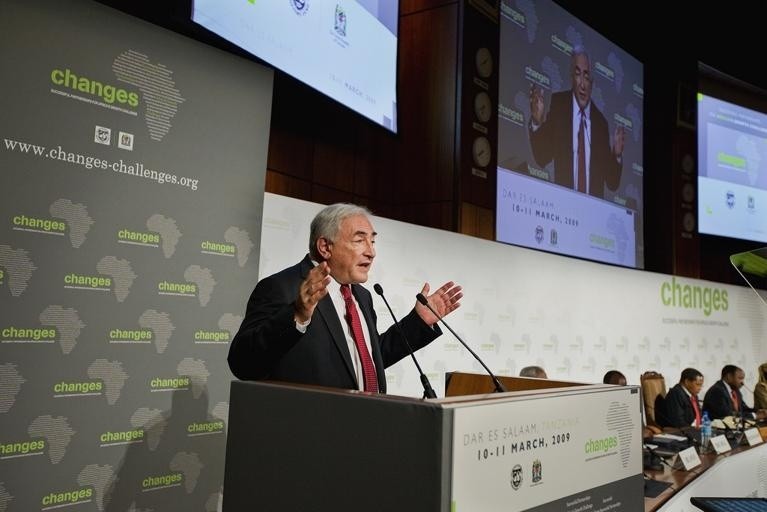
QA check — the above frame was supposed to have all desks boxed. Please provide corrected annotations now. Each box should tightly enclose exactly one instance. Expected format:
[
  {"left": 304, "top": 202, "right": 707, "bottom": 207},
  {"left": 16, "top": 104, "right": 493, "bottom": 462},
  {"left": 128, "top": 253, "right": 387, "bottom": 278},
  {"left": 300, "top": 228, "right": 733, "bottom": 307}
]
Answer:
[{"left": 643, "top": 419, "right": 766, "bottom": 512}]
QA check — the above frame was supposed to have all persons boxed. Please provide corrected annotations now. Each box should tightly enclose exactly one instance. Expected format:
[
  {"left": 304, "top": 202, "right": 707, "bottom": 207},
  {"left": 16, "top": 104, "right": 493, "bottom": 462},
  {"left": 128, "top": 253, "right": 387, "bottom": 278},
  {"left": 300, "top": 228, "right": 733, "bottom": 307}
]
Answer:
[
  {"left": 754, "top": 363, "right": 767, "bottom": 411},
  {"left": 702, "top": 365, "right": 767, "bottom": 423},
  {"left": 520, "top": 366, "right": 548, "bottom": 380},
  {"left": 227, "top": 204, "right": 462, "bottom": 398},
  {"left": 528, "top": 46, "right": 625, "bottom": 200},
  {"left": 662, "top": 368, "right": 704, "bottom": 428},
  {"left": 603, "top": 371, "right": 652, "bottom": 441}
]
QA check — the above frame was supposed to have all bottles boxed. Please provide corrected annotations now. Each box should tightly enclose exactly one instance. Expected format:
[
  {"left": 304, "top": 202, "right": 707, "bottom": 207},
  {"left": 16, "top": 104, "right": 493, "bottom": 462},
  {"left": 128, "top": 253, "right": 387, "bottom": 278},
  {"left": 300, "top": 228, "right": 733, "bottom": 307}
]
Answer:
[
  {"left": 701, "top": 418, "right": 711, "bottom": 448},
  {"left": 702, "top": 411, "right": 711, "bottom": 425}
]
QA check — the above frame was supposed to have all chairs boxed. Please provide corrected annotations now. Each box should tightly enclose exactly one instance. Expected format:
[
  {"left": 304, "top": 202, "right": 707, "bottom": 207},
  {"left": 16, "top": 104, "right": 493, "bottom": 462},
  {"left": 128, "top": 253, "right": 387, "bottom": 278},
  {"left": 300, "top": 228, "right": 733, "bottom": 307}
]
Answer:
[{"left": 640, "top": 371, "right": 667, "bottom": 426}]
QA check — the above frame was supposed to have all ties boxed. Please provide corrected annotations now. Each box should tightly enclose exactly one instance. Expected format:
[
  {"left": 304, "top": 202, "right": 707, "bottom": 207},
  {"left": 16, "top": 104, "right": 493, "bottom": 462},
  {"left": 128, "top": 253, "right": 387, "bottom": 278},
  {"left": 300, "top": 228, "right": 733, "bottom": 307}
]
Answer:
[
  {"left": 340, "top": 284, "right": 378, "bottom": 392},
  {"left": 690, "top": 396, "right": 701, "bottom": 425},
  {"left": 577, "top": 108, "right": 586, "bottom": 193},
  {"left": 732, "top": 391, "right": 739, "bottom": 411}
]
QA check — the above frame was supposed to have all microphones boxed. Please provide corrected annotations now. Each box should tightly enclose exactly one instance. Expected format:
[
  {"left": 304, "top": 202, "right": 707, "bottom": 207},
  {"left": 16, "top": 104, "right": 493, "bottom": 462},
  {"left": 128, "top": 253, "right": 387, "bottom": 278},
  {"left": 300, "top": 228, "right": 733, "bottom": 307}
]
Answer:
[
  {"left": 373, "top": 282, "right": 435, "bottom": 398},
  {"left": 414, "top": 292, "right": 505, "bottom": 392},
  {"left": 739, "top": 382, "right": 767, "bottom": 421},
  {"left": 695, "top": 398, "right": 734, "bottom": 438}
]
